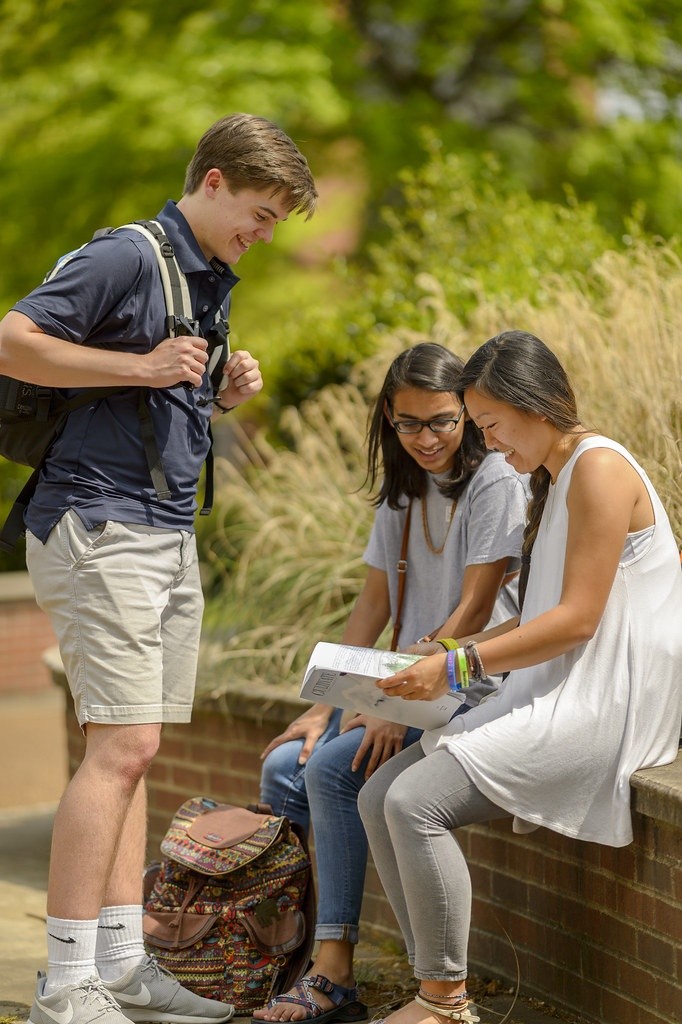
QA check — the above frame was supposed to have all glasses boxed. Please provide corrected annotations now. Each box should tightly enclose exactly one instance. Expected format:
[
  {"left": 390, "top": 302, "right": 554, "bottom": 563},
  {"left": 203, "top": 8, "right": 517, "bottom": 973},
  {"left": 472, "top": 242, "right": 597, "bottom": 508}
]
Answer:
[{"left": 391, "top": 403, "right": 465, "bottom": 434}]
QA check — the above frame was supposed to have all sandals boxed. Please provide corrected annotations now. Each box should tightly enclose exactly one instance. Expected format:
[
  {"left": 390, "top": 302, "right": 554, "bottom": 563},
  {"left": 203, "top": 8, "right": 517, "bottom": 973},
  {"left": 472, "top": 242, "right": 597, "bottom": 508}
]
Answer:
[
  {"left": 371, "top": 991, "right": 481, "bottom": 1024},
  {"left": 249, "top": 970, "right": 369, "bottom": 1024}
]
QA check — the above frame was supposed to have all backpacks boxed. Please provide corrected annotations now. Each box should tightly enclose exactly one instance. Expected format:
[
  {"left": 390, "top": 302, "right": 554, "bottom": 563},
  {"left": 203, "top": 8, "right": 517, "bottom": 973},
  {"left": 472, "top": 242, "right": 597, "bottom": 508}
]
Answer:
[{"left": 1, "top": 219, "right": 236, "bottom": 515}]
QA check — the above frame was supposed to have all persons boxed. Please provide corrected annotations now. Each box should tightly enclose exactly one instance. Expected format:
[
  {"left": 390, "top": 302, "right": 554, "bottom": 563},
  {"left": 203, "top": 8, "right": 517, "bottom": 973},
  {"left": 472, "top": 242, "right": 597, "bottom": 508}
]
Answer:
[
  {"left": 357, "top": 332, "right": 681, "bottom": 1024},
  {"left": 0, "top": 110, "right": 318, "bottom": 1024},
  {"left": 251, "top": 342, "right": 533, "bottom": 1024}
]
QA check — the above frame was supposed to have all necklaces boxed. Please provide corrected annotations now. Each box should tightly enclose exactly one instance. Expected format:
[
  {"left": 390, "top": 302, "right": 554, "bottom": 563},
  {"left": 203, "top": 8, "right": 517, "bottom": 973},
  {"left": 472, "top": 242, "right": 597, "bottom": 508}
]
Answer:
[{"left": 422, "top": 496, "right": 457, "bottom": 554}]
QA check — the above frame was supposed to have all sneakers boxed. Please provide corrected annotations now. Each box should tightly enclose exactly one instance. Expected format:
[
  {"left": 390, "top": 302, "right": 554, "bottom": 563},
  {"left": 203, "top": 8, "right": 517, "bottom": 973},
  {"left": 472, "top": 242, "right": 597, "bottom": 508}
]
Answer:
[
  {"left": 98, "top": 953, "right": 236, "bottom": 1024},
  {"left": 23, "top": 972, "right": 139, "bottom": 1024}
]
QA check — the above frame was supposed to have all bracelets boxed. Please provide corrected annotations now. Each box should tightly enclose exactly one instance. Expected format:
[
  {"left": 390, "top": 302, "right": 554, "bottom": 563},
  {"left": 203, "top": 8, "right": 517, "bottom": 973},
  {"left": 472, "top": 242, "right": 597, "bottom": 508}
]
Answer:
[
  {"left": 214, "top": 402, "right": 239, "bottom": 415},
  {"left": 447, "top": 641, "right": 487, "bottom": 692},
  {"left": 437, "top": 637, "right": 460, "bottom": 653}
]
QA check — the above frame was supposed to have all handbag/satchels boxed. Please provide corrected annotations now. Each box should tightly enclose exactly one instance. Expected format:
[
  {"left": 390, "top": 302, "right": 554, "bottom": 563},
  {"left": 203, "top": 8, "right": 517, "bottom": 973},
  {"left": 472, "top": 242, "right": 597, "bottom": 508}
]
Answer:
[{"left": 145, "top": 794, "right": 319, "bottom": 1019}]
